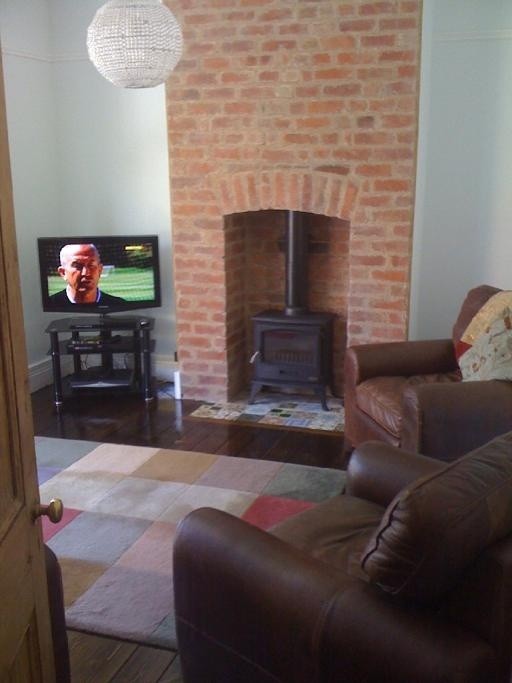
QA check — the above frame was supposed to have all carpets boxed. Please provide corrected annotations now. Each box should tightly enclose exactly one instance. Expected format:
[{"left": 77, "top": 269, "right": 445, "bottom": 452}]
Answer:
[
  {"left": 181, "top": 398, "right": 348, "bottom": 431},
  {"left": 30, "top": 430, "right": 346, "bottom": 647}
]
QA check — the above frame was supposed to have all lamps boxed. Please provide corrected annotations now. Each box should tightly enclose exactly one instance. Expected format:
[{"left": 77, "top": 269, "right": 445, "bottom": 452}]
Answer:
[{"left": 87, "top": 0, "right": 187, "bottom": 88}]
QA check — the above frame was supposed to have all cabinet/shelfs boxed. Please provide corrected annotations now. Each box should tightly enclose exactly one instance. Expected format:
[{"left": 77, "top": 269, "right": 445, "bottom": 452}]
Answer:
[{"left": 47, "top": 314, "right": 161, "bottom": 410}]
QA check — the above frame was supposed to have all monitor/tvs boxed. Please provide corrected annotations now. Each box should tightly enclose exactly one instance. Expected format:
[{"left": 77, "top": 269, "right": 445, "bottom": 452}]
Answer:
[{"left": 37, "top": 236, "right": 162, "bottom": 328}]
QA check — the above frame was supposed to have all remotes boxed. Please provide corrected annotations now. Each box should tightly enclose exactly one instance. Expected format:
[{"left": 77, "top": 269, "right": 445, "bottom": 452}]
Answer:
[{"left": 140, "top": 318, "right": 151, "bottom": 326}]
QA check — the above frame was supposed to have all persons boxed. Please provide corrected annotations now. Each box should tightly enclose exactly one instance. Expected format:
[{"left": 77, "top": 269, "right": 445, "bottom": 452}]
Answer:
[{"left": 47, "top": 244, "right": 131, "bottom": 307}]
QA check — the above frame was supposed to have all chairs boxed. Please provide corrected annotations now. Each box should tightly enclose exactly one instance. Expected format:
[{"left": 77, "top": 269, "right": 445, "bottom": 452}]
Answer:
[
  {"left": 336, "top": 283, "right": 511, "bottom": 461},
  {"left": 170, "top": 440, "right": 512, "bottom": 683}
]
